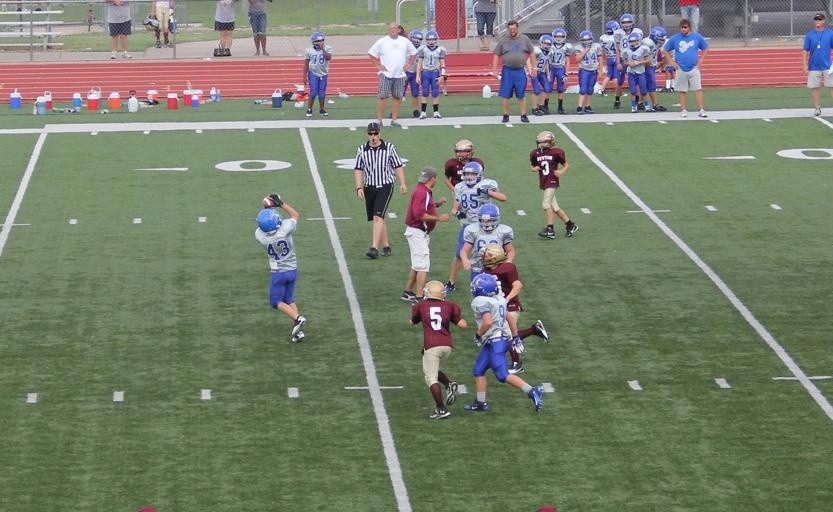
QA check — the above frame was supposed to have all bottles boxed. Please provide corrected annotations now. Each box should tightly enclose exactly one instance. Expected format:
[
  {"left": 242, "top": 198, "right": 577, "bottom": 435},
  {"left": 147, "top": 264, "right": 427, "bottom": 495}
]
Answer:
[
  {"left": 253, "top": 99, "right": 267, "bottom": 105},
  {"left": 33, "top": 101, "right": 37, "bottom": 114},
  {"left": 209, "top": 85, "right": 220, "bottom": 101},
  {"left": 191, "top": 95, "right": 199, "bottom": 107},
  {"left": 482, "top": 84, "right": 492, "bottom": 100}
]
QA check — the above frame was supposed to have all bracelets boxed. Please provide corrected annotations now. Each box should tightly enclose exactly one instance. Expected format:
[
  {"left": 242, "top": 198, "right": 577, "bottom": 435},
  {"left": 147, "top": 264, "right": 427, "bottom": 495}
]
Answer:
[{"left": 356, "top": 188, "right": 361, "bottom": 194}]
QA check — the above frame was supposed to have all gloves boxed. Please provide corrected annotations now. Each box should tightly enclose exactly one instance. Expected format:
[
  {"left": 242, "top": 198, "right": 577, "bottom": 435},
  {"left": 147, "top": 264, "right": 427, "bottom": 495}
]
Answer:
[
  {"left": 269, "top": 192, "right": 284, "bottom": 207},
  {"left": 472, "top": 331, "right": 483, "bottom": 349},
  {"left": 455, "top": 210, "right": 467, "bottom": 221},
  {"left": 559, "top": 72, "right": 569, "bottom": 84},
  {"left": 476, "top": 187, "right": 490, "bottom": 196},
  {"left": 510, "top": 334, "right": 525, "bottom": 355}
]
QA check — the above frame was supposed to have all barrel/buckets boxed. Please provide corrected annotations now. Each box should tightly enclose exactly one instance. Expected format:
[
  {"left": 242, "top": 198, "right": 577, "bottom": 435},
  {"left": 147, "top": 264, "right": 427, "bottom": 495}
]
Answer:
[
  {"left": 272, "top": 88, "right": 283, "bottom": 107},
  {"left": 209, "top": 87, "right": 221, "bottom": 102},
  {"left": 86, "top": 86, "right": 103, "bottom": 110},
  {"left": 108, "top": 91, "right": 121, "bottom": 109},
  {"left": 10, "top": 88, "right": 22, "bottom": 112},
  {"left": 191, "top": 95, "right": 198, "bottom": 107},
  {"left": 167, "top": 93, "right": 179, "bottom": 110},
  {"left": 71, "top": 92, "right": 83, "bottom": 110},
  {"left": 34, "top": 92, "right": 52, "bottom": 116},
  {"left": 128, "top": 94, "right": 139, "bottom": 113},
  {"left": 482, "top": 85, "right": 493, "bottom": 99},
  {"left": 146, "top": 90, "right": 159, "bottom": 103},
  {"left": 297, "top": 89, "right": 304, "bottom": 95}
]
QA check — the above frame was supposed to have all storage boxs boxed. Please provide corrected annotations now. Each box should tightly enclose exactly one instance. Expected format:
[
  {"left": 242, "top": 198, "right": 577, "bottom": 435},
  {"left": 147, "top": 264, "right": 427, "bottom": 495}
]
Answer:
[{"left": 182, "top": 89, "right": 204, "bottom": 106}]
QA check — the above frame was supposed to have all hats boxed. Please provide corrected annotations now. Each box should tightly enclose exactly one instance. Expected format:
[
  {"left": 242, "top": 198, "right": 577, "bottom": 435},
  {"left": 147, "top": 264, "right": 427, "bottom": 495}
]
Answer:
[
  {"left": 813, "top": 11, "right": 826, "bottom": 21},
  {"left": 417, "top": 165, "right": 439, "bottom": 184},
  {"left": 366, "top": 122, "right": 380, "bottom": 132}
]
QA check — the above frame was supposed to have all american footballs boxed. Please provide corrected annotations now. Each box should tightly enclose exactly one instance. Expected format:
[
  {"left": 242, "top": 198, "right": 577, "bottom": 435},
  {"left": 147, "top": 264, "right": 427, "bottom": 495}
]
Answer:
[{"left": 263, "top": 193, "right": 280, "bottom": 209}]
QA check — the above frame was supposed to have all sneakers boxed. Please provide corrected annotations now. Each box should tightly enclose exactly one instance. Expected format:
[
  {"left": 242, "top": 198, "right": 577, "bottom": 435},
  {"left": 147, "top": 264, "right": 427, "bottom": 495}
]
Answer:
[
  {"left": 527, "top": 383, "right": 544, "bottom": 412},
  {"left": 443, "top": 281, "right": 457, "bottom": 295},
  {"left": 374, "top": 90, "right": 449, "bottom": 129},
  {"left": 538, "top": 222, "right": 581, "bottom": 240},
  {"left": 813, "top": 104, "right": 823, "bottom": 117},
  {"left": 365, "top": 247, "right": 392, "bottom": 259},
  {"left": 495, "top": 85, "right": 712, "bottom": 124},
  {"left": 400, "top": 289, "right": 423, "bottom": 307},
  {"left": 154, "top": 40, "right": 174, "bottom": 49},
  {"left": 290, "top": 314, "right": 308, "bottom": 343},
  {"left": 108, "top": 50, "right": 131, "bottom": 60},
  {"left": 429, "top": 380, "right": 489, "bottom": 420},
  {"left": 304, "top": 108, "right": 329, "bottom": 119},
  {"left": 507, "top": 360, "right": 526, "bottom": 375},
  {"left": 531, "top": 319, "right": 549, "bottom": 343}
]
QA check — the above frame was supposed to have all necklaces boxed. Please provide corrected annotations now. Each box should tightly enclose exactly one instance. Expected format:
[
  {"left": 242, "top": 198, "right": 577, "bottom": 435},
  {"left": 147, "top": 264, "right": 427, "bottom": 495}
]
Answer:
[{"left": 814, "top": 29, "right": 823, "bottom": 49}]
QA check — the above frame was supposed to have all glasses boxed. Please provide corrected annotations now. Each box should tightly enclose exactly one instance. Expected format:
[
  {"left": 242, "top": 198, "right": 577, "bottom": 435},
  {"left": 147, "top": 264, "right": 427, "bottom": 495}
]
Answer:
[
  {"left": 367, "top": 131, "right": 379, "bottom": 136},
  {"left": 680, "top": 25, "right": 689, "bottom": 29}
]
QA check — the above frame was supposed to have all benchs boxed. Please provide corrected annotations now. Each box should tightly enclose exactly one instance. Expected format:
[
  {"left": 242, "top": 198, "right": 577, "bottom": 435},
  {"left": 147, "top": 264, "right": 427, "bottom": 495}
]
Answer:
[{"left": 0, "top": 0, "right": 64, "bottom": 51}]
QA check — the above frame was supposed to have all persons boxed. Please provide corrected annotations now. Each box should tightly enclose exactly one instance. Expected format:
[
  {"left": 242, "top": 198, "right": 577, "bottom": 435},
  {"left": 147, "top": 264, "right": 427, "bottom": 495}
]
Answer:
[
  {"left": 802, "top": 13, "right": 833, "bottom": 116},
  {"left": 530, "top": 131, "right": 578, "bottom": 238},
  {"left": 493, "top": 15, "right": 708, "bottom": 123},
  {"left": 151, "top": 0, "right": 174, "bottom": 48},
  {"left": 214, "top": 0, "right": 239, "bottom": 49},
  {"left": 303, "top": 32, "right": 332, "bottom": 116},
  {"left": 248, "top": 0, "right": 273, "bottom": 55},
  {"left": 402, "top": 139, "right": 548, "bottom": 418},
  {"left": 473, "top": 0, "right": 498, "bottom": 51},
  {"left": 104, "top": 0, "right": 132, "bottom": 59},
  {"left": 368, "top": 22, "right": 447, "bottom": 125},
  {"left": 255, "top": 194, "right": 306, "bottom": 342},
  {"left": 679, "top": 0, "right": 699, "bottom": 33},
  {"left": 354, "top": 122, "right": 407, "bottom": 259}
]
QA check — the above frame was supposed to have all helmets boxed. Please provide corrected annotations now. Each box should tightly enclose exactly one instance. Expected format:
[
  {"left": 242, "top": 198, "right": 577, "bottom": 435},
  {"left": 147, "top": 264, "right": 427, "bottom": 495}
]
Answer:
[
  {"left": 255, "top": 207, "right": 283, "bottom": 236},
  {"left": 578, "top": 30, "right": 595, "bottom": 49},
  {"left": 454, "top": 138, "right": 475, "bottom": 163},
  {"left": 648, "top": 25, "right": 667, "bottom": 46},
  {"left": 604, "top": 13, "right": 635, "bottom": 35},
  {"left": 310, "top": 31, "right": 326, "bottom": 51},
  {"left": 422, "top": 280, "right": 447, "bottom": 302},
  {"left": 462, "top": 161, "right": 485, "bottom": 185},
  {"left": 539, "top": 27, "right": 569, "bottom": 52},
  {"left": 408, "top": 28, "right": 440, "bottom": 49},
  {"left": 628, "top": 32, "right": 642, "bottom": 52},
  {"left": 470, "top": 272, "right": 499, "bottom": 297},
  {"left": 478, "top": 242, "right": 508, "bottom": 269},
  {"left": 536, "top": 130, "right": 555, "bottom": 152},
  {"left": 478, "top": 203, "right": 500, "bottom": 234}
]
